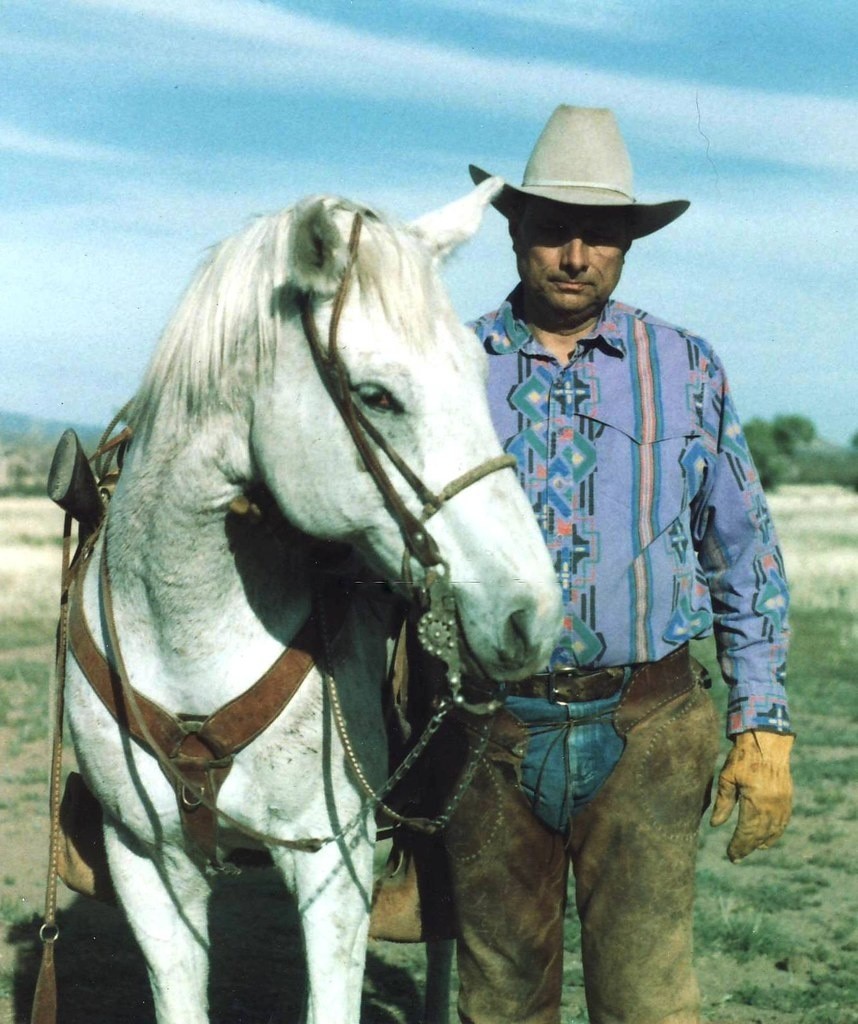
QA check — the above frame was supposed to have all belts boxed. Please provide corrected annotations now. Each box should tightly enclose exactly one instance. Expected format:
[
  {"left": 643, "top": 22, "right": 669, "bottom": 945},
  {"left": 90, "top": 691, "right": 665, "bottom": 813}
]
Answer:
[{"left": 463, "top": 664, "right": 637, "bottom": 702}]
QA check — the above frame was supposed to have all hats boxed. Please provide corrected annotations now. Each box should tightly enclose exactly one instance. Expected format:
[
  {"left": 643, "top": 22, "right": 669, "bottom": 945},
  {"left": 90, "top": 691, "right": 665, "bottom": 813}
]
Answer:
[{"left": 468, "top": 104, "right": 690, "bottom": 239}]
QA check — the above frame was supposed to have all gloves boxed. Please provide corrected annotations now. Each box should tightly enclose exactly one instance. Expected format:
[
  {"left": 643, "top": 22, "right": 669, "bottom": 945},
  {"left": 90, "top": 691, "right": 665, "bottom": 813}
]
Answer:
[{"left": 710, "top": 728, "right": 798, "bottom": 862}]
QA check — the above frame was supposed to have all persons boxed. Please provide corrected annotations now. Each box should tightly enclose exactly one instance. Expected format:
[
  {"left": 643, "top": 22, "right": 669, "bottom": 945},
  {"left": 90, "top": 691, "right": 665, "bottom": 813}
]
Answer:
[{"left": 383, "top": 103, "right": 796, "bottom": 1023}]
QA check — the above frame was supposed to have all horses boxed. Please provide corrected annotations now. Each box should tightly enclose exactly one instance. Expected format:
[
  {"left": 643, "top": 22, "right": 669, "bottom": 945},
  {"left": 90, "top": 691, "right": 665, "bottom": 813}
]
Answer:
[{"left": 60, "top": 179, "right": 563, "bottom": 1024}]
